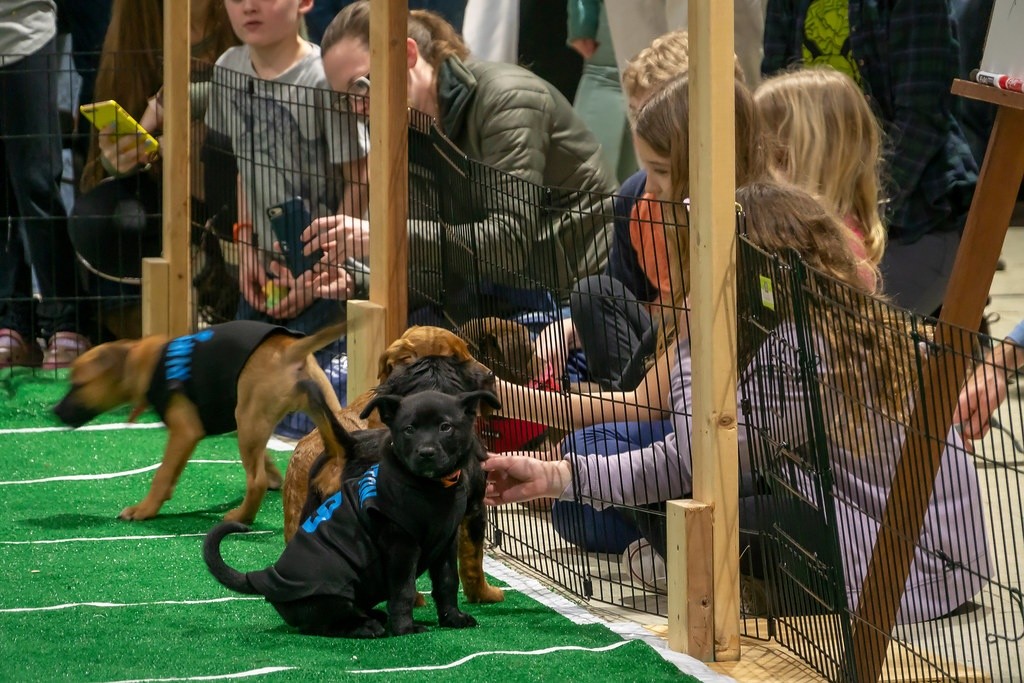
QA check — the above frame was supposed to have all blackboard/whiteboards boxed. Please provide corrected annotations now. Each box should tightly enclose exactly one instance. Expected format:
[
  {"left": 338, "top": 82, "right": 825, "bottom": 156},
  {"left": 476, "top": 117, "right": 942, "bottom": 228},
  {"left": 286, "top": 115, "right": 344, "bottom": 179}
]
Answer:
[{"left": 979, "top": 0, "right": 1024, "bottom": 94}]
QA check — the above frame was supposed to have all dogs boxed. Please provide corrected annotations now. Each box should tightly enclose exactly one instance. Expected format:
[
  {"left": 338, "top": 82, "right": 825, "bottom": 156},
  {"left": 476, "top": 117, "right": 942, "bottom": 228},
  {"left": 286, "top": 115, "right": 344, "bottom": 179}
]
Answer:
[
  {"left": 200, "top": 316, "right": 572, "bottom": 639},
  {"left": 53, "top": 319, "right": 348, "bottom": 534}
]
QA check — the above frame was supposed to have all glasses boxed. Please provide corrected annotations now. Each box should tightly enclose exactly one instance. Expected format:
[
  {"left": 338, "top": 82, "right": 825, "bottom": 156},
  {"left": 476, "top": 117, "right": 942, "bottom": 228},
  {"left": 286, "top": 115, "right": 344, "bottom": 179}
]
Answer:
[{"left": 333, "top": 75, "right": 372, "bottom": 113}]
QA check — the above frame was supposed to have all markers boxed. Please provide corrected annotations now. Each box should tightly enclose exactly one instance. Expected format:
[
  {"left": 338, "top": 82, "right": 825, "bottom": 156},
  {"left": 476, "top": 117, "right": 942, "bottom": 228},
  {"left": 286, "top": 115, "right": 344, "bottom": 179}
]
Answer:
[
  {"left": 970, "top": 68, "right": 1006, "bottom": 89},
  {"left": 998, "top": 76, "right": 1024, "bottom": 92}
]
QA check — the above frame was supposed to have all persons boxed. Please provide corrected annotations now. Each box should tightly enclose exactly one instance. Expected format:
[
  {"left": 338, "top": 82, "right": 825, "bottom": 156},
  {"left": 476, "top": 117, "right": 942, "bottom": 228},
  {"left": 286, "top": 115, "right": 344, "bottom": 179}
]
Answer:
[
  {"left": 474, "top": 180, "right": 993, "bottom": 625},
  {"left": 750, "top": 65, "right": 887, "bottom": 294},
  {"left": 567, "top": 0, "right": 746, "bottom": 185},
  {"left": 475, "top": 67, "right": 779, "bottom": 555},
  {"left": 0, "top": 0, "right": 96, "bottom": 368},
  {"left": 757, "top": 0, "right": 980, "bottom": 248},
  {"left": 204, "top": 0, "right": 371, "bottom": 437},
  {"left": 569, "top": 30, "right": 746, "bottom": 393},
  {"left": 69, "top": 0, "right": 245, "bottom": 344},
  {"left": 275, "top": 0, "right": 620, "bottom": 383}
]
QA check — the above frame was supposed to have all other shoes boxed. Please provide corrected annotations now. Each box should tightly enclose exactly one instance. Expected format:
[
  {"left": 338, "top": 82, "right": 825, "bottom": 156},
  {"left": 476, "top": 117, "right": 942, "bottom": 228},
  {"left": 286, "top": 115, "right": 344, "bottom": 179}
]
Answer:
[{"left": 1, "top": 326, "right": 93, "bottom": 368}]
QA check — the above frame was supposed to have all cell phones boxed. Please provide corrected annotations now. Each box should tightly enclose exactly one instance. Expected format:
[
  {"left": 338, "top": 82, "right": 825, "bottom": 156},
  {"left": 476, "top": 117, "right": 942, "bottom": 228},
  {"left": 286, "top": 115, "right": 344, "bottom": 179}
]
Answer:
[
  {"left": 79, "top": 99, "right": 159, "bottom": 163},
  {"left": 267, "top": 195, "right": 326, "bottom": 280}
]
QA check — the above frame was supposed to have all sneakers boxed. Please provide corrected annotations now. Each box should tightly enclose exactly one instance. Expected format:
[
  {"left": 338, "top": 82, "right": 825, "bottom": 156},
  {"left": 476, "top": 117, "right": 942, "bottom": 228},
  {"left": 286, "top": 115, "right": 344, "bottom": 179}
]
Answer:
[{"left": 623, "top": 538, "right": 774, "bottom": 616}]
